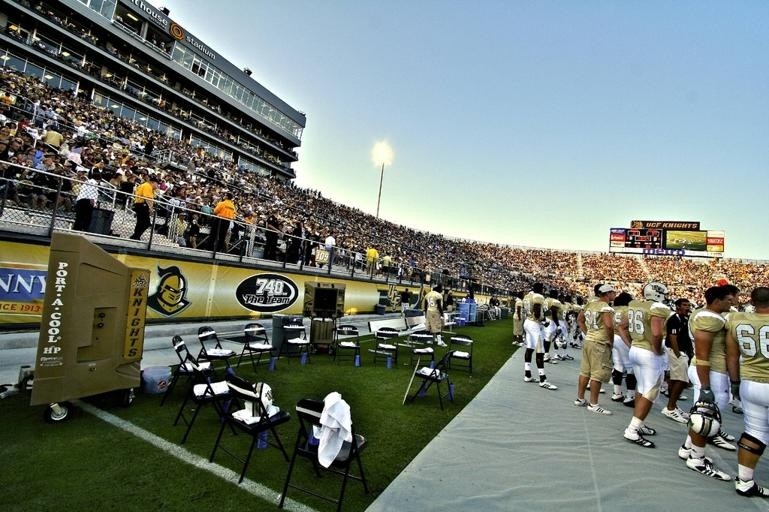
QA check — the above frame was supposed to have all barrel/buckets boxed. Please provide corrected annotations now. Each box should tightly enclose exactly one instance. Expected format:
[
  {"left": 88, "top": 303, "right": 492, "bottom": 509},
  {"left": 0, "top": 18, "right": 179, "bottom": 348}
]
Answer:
[{"left": 141, "top": 365, "right": 172, "bottom": 394}]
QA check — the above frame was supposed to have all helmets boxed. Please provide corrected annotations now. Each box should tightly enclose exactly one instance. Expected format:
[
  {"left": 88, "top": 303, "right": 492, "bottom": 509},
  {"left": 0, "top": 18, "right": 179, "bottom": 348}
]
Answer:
[
  {"left": 643, "top": 282, "right": 670, "bottom": 303},
  {"left": 690, "top": 403, "right": 723, "bottom": 438}
]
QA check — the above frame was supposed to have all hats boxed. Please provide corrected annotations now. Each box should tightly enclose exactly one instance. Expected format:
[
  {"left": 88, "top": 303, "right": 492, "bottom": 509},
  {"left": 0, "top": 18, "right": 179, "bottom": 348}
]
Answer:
[{"left": 599, "top": 285, "right": 618, "bottom": 294}]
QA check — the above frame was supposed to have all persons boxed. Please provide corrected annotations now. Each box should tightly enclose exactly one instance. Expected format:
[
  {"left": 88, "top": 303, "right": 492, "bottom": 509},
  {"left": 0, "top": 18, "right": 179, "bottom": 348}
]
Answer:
[
  {"left": 616, "top": 281, "right": 671, "bottom": 448},
  {"left": 0, "top": 0, "right": 295, "bottom": 269},
  {"left": 511, "top": 244, "right": 768, "bottom": 311},
  {"left": 513, "top": 284, "right": 585, "bottom": 389},
  {"left": 424, "top": 285, "right": 448, "bottom": 348},
  {"left": 660, "top": 298, "right": 694, "bottom": 424},
  {"left": 724, "top": 286, "right": 769, "bottom": 497},
  {"left": 707, "top": 283, "right": 737, "bottom": 451},
  {"left": 575, "top": 284, "right": 636, "bottom": 414},
  {"left": 678, "top": 285, "right": 733, "bottom": 481},
  {"left": 293, "top": 180, "right": 514, "bottom": 327}
]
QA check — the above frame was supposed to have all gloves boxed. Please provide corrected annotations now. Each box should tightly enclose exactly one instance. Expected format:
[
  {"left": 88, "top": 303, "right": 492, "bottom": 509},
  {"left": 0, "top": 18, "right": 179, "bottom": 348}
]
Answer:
[{"left": 697, "top": 389, "right": 716, "bottom": 402}]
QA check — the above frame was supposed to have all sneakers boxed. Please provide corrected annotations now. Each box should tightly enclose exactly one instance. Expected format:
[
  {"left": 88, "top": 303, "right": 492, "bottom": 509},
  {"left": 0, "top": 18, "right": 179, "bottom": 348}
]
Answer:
[
  {"left": 575, "top": 397, "right": 612, "bottom": 415},
  {"left": 730, "top": 405, "right": 742, "bottom": 414},
  {"left": 709, "top": 429, "right": 736, "bottom": 451},
  {"left": 525, "top": 376, "right": 557, "bottom": 391},
  {"left": 735, "top": 478, "right": 769, "bottom": 496},
  {"left": 432, "top": 338, "right": 451, "bottom": 348},
  {"left": 678, "top": 446, "right": 731, "bottom": 482},
  {"left": 662, "top": 405, "right": 691, "bottom": 423},
  {"left": 625, "top": 423, "right": 657, "bottom": 450},
  {"left": 612, "top": 391, "right": 635, "bottom": 407},
  {"left": 587, "top": 383, "right": 605, "bottom": 394},
  {"left": 514, "top": 339, "right": 583, "bottom": 365},
  {"left": 660, "top": 384, "right": 688, "bottom": 401}
]
